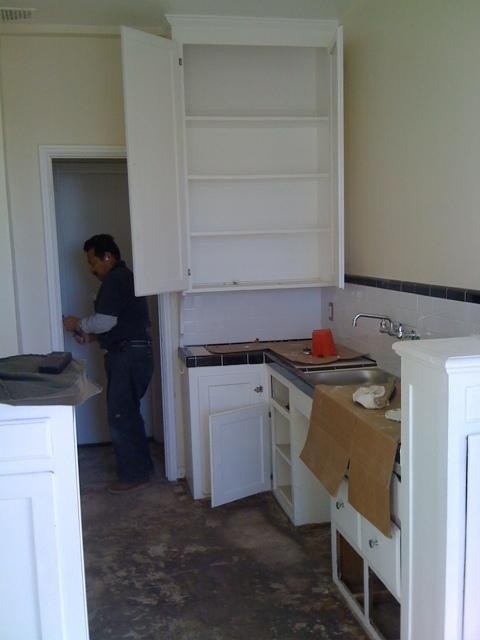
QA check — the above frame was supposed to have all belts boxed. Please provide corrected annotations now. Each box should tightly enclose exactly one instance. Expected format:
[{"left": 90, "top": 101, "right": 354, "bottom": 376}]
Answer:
[{"left": 106, "top": 340, "right": 151, "bottom": 350}]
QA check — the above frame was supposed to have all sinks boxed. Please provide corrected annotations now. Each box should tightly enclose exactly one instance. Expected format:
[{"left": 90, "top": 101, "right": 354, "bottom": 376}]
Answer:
[
  {"left": 305, "top": 367, "right": 402, "bottom": 387},
  {"left": 352, "top": 314, "right": 394, "bottom": 336}
]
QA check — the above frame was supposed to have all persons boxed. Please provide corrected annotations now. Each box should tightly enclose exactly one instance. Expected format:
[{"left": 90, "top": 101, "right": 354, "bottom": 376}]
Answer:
[{"left": 62, "top": 233, "right": 154, "bottom": 492}]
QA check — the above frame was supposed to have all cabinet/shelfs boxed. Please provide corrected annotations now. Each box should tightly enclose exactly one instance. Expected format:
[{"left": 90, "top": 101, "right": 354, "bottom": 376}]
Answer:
[
  {"left": 330, "top": 459, "right": 402, "bottom": 640},
  {"left": 118, "top": 16, "right": 346, "bottom": 297},
  {"left": 205, "top": 366, "right": 294, "bottom": 520},
  {"left": 187, "top": 365, "right": 267, "bottom": 501}
]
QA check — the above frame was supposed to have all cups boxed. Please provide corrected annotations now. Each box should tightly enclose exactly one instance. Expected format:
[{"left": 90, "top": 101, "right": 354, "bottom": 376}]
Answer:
[{"left": 311, "top": 328, "right": 338, "bottom": 357}]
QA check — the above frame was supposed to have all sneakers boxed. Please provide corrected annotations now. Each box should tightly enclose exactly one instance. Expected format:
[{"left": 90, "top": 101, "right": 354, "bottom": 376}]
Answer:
[{"left": 105, "top": 475, "right": 152, "bottom": 494}]
